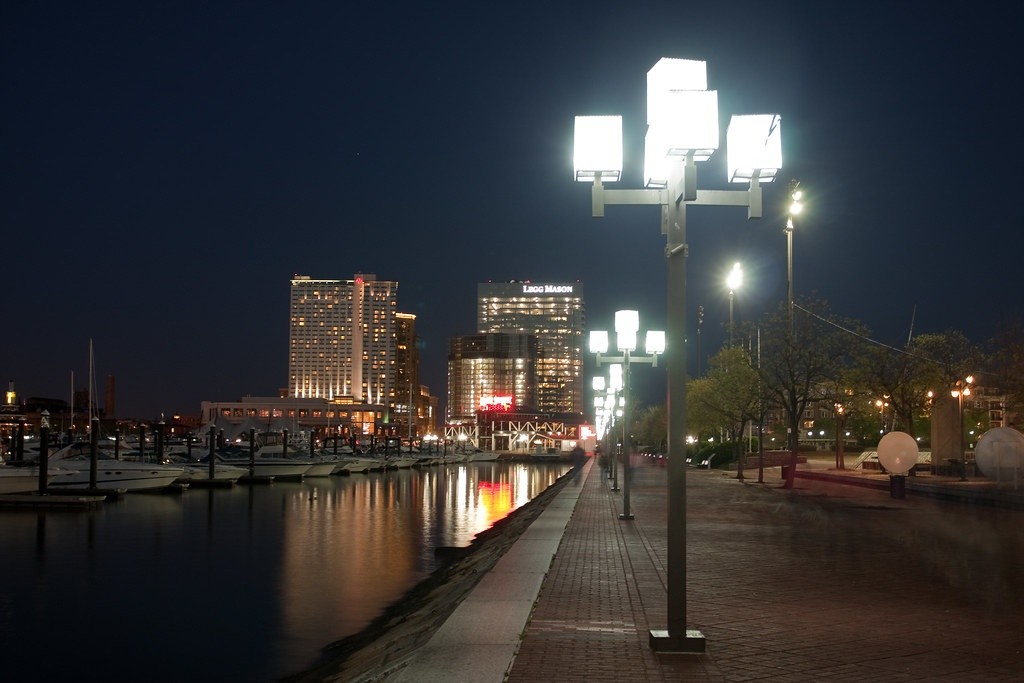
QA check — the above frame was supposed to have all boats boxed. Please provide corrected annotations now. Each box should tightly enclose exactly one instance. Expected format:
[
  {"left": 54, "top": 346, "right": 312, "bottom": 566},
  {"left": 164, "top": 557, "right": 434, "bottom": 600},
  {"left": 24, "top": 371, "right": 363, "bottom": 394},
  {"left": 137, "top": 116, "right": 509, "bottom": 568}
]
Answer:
[{"left": 0, "top": 431, "right": 501, "bottom": 493}]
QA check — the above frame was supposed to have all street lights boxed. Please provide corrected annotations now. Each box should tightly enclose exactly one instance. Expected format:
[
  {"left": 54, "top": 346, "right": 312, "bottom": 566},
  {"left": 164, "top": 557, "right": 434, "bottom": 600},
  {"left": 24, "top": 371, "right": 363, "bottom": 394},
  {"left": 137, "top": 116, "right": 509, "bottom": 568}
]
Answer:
[
  {"left": 572, "top": 55, "right": 784, "bottom": 652},
  {"left": 950, "top": 374, "right": 975, "bottom": 483},
  {"left": 592, "top": 364, "right": 623, "bottom": 492},
  {"left": 589, "top": 310, "right": 667, "bottom": 519},
  {"left": 725, "top": 261, "right": 746, "bottom": 479},
  {"left": 875, "top": 394, "right": 890, "bottom": 436},
  {"left": 784, "top": 178, "right": 804, "bottom": 495}
]
